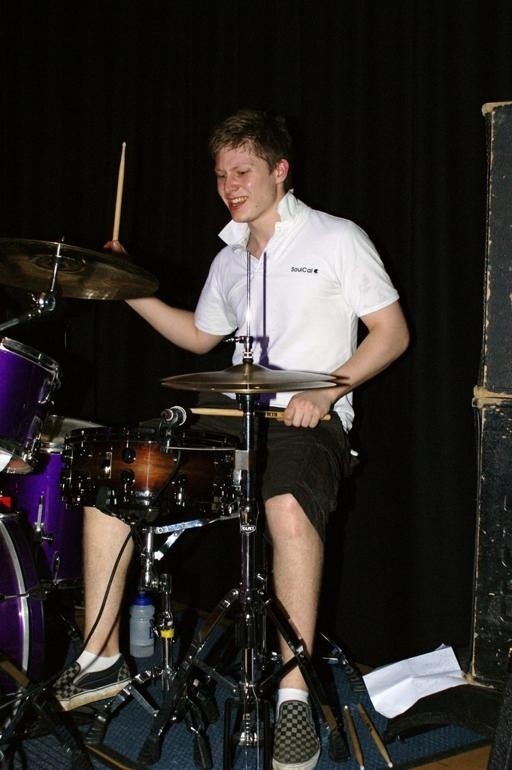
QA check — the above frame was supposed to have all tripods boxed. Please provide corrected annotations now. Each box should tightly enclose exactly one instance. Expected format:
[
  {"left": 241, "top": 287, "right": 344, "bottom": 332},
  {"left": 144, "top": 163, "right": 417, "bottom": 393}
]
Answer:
[
  {"left": 137, "top": 411, "right": 352, "bottom": 770},
  {"left": 83, "top": 533, "right": 221, "bottom": 770}
]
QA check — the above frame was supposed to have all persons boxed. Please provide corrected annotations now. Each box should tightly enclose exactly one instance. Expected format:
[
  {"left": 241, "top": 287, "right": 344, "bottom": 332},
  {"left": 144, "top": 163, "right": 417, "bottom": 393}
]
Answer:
[{"left": 52, "top": 107, "right": 413, "bottom": 770}]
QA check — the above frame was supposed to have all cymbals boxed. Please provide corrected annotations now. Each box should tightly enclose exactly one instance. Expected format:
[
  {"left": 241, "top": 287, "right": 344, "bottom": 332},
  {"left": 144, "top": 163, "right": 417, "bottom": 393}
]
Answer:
[
  {"left": 161, "top": 363, "right": 336, "bottom": 394},
  {"left": 0, "top": 236, "right": 161, "bottom": 301}
]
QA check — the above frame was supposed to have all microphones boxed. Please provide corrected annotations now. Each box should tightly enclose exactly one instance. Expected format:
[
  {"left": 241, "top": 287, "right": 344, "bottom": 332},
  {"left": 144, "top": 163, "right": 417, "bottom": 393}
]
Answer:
[{"left": 160, "top": 404, "right": 192, "bottom": 426}]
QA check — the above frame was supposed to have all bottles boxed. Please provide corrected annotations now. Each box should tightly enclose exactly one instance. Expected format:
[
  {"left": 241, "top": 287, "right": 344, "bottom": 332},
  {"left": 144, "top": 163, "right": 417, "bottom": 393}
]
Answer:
[{"left": 127, "top": 588, "right": 156, "bottom": 658}]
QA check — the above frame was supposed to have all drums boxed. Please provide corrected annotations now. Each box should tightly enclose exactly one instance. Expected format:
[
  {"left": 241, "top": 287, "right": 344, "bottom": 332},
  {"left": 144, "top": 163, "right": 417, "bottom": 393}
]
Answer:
[
  {"left": 0, "top": 419, "right": 107, "bottom": 593},
  {"left": 0, "top": 491, "right": 48, "bottom": 747},
  {"left": 60, "top": 423, "right": 240, "bottom": 526},
  {"left": 0, "top": 336, "right": 63, "bottom": 477}
]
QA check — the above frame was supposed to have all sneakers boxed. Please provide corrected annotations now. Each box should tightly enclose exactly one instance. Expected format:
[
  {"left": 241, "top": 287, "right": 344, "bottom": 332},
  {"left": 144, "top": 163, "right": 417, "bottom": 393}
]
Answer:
[
  {"left": 271, "top": 699, "right": 321, "bottom": 770},
  {"left": 54, "top": 653, "right": 133, "bottom": 709}
]
